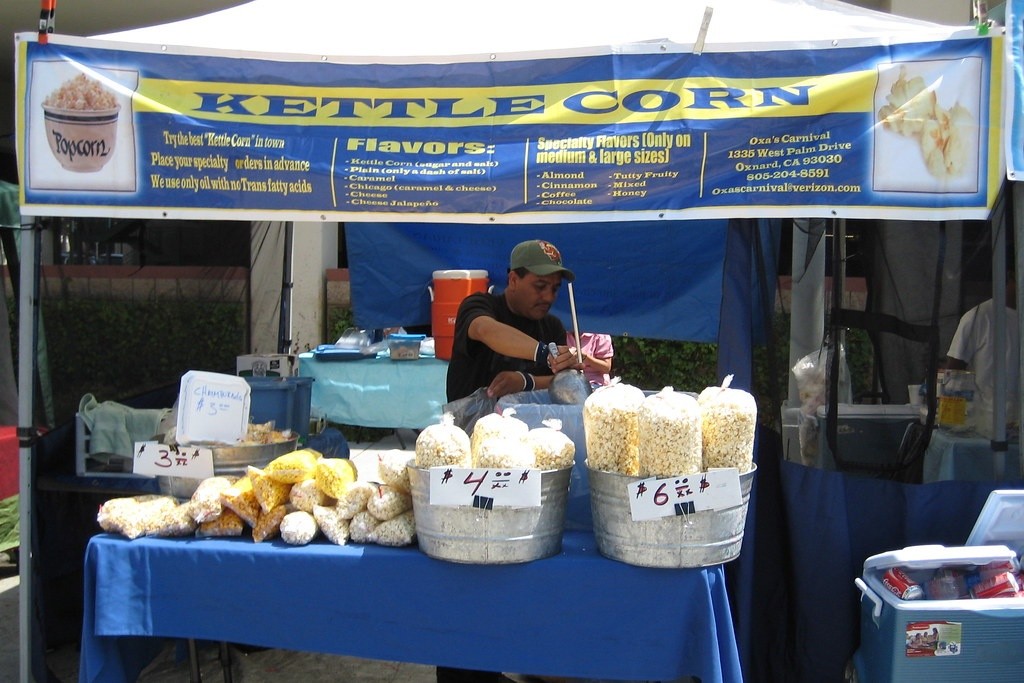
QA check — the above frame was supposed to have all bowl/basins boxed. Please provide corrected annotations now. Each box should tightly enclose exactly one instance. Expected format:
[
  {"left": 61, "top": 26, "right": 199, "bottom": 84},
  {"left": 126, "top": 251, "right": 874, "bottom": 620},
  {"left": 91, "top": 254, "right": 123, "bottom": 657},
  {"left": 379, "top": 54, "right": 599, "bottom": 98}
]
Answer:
[{"left": 42, "top": 103, "right": 118, "bottom": 174}]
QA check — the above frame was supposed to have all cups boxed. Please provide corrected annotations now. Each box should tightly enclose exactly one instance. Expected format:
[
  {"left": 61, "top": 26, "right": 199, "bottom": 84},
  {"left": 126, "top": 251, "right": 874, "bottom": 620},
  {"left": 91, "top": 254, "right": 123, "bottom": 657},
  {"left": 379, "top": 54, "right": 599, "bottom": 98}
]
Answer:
[{"left": 908, "top": 384, "right": 923, "bottom": 408}]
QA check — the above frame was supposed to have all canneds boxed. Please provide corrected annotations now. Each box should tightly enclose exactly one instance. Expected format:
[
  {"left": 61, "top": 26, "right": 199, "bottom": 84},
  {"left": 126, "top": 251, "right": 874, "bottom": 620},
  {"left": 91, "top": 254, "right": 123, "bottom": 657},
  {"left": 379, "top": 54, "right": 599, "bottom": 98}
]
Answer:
[
  {"left": 881, "top": 567, "right": 924, "bottom": 600},
  {"left": 968, "top": 558, "right": 1024, "bottom": 599}
]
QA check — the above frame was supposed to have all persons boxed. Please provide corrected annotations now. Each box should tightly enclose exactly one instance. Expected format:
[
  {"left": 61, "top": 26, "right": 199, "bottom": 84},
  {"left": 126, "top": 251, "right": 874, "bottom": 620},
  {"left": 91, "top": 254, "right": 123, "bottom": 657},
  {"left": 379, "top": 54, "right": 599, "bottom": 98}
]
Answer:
[
  {"left": 947, "top": 241, "right": 1020, "bottom": 445},
  {"left": 447, "top": 240, "right": 585, "bottom": 437},
  {"left": 567, "top": 331, "right": 614, "bottom": 392}
]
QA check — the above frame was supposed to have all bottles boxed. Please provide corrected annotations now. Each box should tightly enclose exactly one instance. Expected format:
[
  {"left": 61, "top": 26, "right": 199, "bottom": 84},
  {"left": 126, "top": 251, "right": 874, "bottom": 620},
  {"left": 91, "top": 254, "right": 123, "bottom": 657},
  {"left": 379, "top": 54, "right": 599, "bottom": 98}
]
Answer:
[{"left": 938, "top": 368, "right": 979, "bottom": 434}]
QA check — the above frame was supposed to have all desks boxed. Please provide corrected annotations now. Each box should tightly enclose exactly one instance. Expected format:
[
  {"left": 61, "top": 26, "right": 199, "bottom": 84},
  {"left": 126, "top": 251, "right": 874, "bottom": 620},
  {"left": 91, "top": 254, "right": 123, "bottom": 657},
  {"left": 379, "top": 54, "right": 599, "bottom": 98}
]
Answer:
[
  {"left": 300, "top": 346, "right": 448, "bottom": 451},
  {"left": 77, "top": 530, "right": 746, "bottom": 683}
]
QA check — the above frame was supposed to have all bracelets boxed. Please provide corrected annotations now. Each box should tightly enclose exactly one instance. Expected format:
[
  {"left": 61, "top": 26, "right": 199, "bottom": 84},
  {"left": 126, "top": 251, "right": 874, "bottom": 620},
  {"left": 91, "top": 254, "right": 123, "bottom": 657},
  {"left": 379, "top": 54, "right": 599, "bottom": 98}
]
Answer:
[
  {"left": 582, "top": 355, "right": 587, "bottom": 360},
  {"left": 517, "top": 370, "right": 535, "bottom": 391},
  {"left": 534, "top": 341, "right": 548, "bottom": 372}
]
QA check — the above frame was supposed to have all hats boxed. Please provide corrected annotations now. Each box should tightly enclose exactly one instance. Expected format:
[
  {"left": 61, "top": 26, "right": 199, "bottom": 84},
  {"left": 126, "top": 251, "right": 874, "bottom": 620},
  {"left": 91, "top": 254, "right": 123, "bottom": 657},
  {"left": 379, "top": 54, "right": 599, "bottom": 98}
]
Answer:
[{"left": 511, "top": 240, "right": 575, "bottom": 282}]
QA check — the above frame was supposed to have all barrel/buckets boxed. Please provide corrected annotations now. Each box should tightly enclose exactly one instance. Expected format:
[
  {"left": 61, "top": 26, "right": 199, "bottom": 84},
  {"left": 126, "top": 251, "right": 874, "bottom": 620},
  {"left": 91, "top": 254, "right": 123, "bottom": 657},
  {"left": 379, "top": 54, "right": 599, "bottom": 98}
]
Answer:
[
  {"left": 408, "top": 462, "right": 574, "bottom": 564},
  {"left": 584, "top": 462, "right": 757, "bottom": 567},
  {"left": 149, "top": 430, "right": 299, "bottom": 503},
  {"left": 429, "top": 270, "right": 489, "bottom": 359}
]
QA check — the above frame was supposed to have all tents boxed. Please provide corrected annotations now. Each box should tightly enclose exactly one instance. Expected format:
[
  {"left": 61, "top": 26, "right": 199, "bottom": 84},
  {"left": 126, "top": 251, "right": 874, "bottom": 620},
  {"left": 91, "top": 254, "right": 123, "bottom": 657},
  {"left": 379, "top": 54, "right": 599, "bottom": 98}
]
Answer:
[{"left": 14, "top": 0, "right": 1024, "bottom": 683}]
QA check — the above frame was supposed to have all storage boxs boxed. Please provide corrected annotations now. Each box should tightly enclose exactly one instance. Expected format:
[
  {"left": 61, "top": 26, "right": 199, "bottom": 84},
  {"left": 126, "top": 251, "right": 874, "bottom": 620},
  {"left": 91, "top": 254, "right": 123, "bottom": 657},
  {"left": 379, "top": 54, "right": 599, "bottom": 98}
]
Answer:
[
  {"left": 246, "top": 376, "right": 316, "bottom": 446},
  {"left": 855, "top": 543, "right": 1024, "bottom": 683},
  {"left": 804, "top": 405, "right": 921, "bottom": 484}
]
